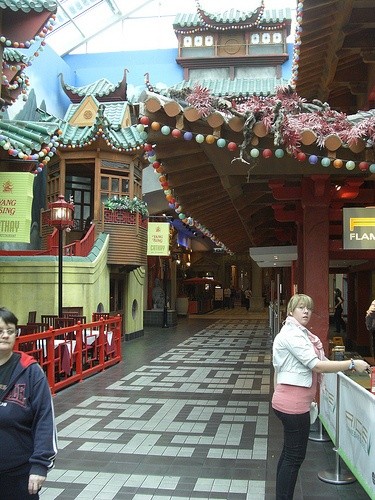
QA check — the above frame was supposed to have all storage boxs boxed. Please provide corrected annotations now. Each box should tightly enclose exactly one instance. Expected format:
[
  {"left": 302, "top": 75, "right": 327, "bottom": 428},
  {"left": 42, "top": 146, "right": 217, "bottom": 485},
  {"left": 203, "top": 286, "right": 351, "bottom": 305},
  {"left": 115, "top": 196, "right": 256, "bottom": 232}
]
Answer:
[
  {"left": 176, "top": 297, "right": 190, "bottom": 312},
  {"left": 189, "top": 301, "right": 201, "bottom": 313}
]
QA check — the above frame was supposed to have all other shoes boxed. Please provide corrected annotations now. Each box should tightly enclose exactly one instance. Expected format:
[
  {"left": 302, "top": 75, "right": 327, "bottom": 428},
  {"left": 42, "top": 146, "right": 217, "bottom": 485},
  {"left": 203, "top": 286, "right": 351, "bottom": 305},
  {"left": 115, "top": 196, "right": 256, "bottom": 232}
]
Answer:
[
  {"left": 344, "top": 328, "right": 346, "bottom": 333},
  {"left": 333, "top": 330, "right": 341, "bottom": 333}
]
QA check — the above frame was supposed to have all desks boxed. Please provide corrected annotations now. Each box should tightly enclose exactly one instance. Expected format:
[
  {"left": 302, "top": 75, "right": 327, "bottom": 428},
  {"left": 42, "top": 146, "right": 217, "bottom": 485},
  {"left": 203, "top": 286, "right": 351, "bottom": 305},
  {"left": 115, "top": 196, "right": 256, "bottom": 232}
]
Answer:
[
  {"left": 37, "top": 339, "right": 77, "bottom": 375},
  {"left": 64, "top": 329, "right": 113, "bottom": 354}
]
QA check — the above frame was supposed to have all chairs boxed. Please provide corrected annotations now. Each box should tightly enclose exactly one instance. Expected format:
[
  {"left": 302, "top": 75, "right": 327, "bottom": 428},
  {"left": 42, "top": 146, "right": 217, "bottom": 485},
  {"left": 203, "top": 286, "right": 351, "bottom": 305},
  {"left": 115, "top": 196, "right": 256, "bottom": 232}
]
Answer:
[{"left": 17, "top": 311, "right": 110, "bottom": 382}]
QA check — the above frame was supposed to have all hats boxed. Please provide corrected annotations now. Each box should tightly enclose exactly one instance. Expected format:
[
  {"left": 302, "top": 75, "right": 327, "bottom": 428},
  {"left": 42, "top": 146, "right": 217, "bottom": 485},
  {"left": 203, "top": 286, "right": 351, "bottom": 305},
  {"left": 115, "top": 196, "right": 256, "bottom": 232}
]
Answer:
[{"left": 334, "top": 288, "right": 340, "bottom": 294}]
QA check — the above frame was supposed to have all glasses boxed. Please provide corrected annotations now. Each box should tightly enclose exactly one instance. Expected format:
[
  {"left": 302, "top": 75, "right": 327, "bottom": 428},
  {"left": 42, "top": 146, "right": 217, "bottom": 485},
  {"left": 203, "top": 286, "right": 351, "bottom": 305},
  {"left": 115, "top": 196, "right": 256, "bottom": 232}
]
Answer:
[
  {"left": 0, "top": 328, "right": 18, "bottom": 335},
  {"left": 295, "top": 306, "right": 312, "bottom": 311}
]
